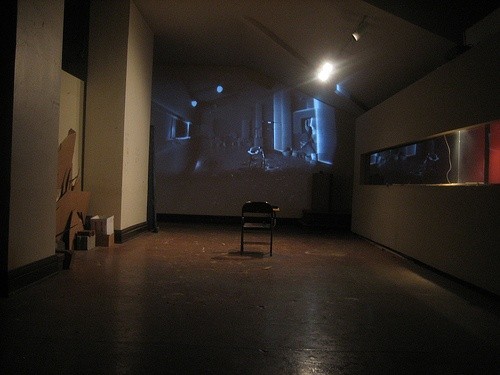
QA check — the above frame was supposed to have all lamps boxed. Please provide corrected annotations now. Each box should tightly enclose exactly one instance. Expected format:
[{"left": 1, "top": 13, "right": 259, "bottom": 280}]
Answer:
[{"left": 351, "top": 15, "right": 372, "bottom": 41}]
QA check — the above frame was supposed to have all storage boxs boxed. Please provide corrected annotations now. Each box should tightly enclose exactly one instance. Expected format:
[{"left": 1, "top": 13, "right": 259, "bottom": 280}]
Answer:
[
  {"left": 88, "top": 214, "right": 114, "bottom": 235},
  {"left": 73, "top": 230, "right": 97, "bottom": 250}
]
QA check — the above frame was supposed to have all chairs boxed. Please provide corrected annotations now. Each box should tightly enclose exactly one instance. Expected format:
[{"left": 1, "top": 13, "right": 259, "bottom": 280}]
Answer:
[{"left": 239, "top": 201, "right": 277, "bottom": 257}]
